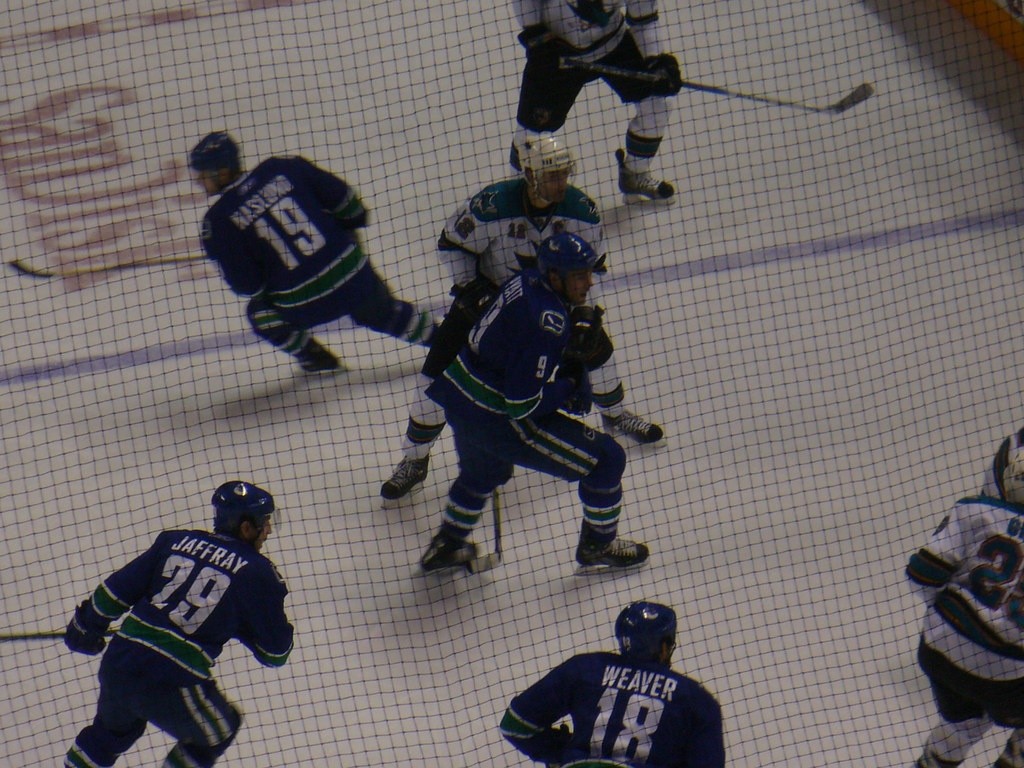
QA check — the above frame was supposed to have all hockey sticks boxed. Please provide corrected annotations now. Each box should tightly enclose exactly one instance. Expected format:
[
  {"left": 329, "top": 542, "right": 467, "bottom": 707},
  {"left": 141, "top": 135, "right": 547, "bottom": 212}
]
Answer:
[
  {"left": 459, "top": 487, "right": 508, "bottom": 576},
  {"left": 0, "top": 626, "right": 121, "bottom": 643},
  {"left": 7, "top": 244, "right": 216, "bottom": 279},
  {"left": 550, "top": 39, "right": 875, "bottom": 114}
]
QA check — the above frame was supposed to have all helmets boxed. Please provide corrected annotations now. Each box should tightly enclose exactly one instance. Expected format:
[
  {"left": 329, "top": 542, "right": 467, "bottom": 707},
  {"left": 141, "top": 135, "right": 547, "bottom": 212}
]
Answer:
[
  {"left": 518, "top": 135, "right": 575, "bottom": 186},
  {"left": 212, "top": 481, "right": 274, "bottom": 531},
  {"left": 537, "top": 232, "right": 597, "bottom": 282},
  {"left": 615, "top": 601, "right": 676, "bottom": 657},
  {"left": 1004, "top": 446, "right": 1024, "bottom": 502},
  {"left": 190, "top": 131, "right": 240, "bottom": 177}
]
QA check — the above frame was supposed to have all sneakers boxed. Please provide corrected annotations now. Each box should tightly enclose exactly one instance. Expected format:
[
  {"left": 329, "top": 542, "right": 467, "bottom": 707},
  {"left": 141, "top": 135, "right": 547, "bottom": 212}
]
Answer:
[
  {"left": 420, "top": 521, "right": 477, "bottom": 570},
  {"left": 615, "top": 148, "right": 676, "bottom": 206},
  {"left": 602, "top": 409, "right": 664, "bottom": 448},
  {"left": 576, "top": 518, "right": 649, "bottom": 576},
  {"left": 381, "top": 455, "right": 429, "bottom": 509},
  {"left": 301, "top": 338, "right": 339, "bottom": 372}
]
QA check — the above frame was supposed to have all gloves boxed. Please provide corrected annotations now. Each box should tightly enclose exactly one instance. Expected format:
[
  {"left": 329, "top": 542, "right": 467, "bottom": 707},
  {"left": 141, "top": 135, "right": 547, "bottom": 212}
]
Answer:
[
  {"left": 555, "top": 362, "right": 592, "bottom": 415},
  {"left": 518, "top": 23, "right": 583, "bottom": 75},
  {"left": 65, "top": 605, "right": 107, "bottom": 655},
  {"left": 450, "top": 277, "right": 496, "bottom": 321},
  {"left": 637, "top": 55, "right": 682, "bottom": 97},
  {"left": 571, "top": 306, "right": 604, "bottom": 358}
]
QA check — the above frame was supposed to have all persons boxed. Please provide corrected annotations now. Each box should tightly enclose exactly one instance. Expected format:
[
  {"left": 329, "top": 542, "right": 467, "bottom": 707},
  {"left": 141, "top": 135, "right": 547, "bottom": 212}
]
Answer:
[
  {"left": 379, "top": 135, "right": 664, "bottom": 505},
  {"left": 500, "top": 601, "right": 726, "bottom": 768},
  {"left": 190, "top": 132, "right": 439, "bottom": 373},
  {"left": 509, "top": 0, "right": 681, "bottom": 205},
  {"left": 904, "top": 427, "right": 1024, "bottom": 768},
  {"left": 422, "top": 232, "right": 649, "bottom": 584},
  {"left": 61, "top": 480, "right": 294, "bottom": 768}
]
